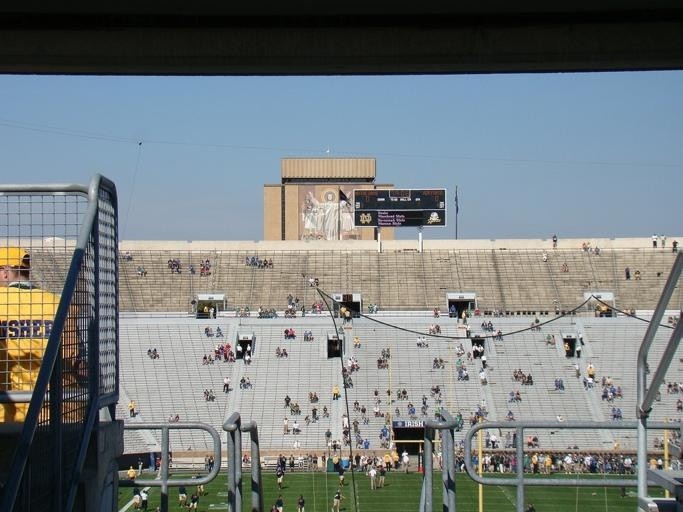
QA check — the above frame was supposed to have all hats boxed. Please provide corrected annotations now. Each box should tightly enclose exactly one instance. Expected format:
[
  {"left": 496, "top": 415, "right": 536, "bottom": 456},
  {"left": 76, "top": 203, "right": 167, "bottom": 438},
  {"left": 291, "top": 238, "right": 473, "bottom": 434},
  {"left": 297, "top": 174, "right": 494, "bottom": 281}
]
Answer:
[{"left": 0, "top": 246, "right": 31, "bottom": 269}]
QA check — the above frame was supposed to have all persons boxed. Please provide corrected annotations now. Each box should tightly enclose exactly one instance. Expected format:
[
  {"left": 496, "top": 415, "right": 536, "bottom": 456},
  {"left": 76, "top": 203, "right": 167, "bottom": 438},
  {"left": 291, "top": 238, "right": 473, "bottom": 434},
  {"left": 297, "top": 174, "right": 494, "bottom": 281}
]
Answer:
[
  {"left": 0, "top": 245, "right": 90, "bottom": 424},
  {"left": 661, "top": 235, "right": 667, "bottom": 248},
  {"left": 121, "top": 248, "right": 416, "bottom": 511},
  {"left": 551, "top": 234, "right": 557, "bottom": 250},
  {"left": 651, "top": 234, "right": 658, "bottom": 248},
  {"left": 308, "top": 190, "right": 354, "bottom": 240},
  {"left": 417, "top": 241, "right": 683, "bottom": 512}
]
